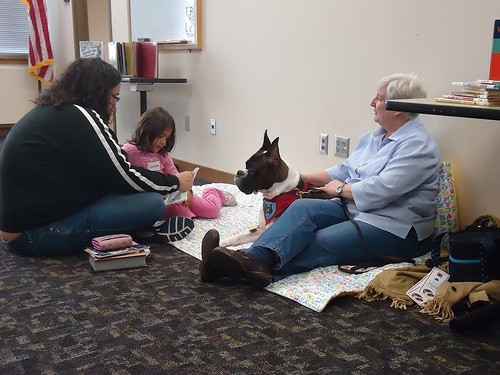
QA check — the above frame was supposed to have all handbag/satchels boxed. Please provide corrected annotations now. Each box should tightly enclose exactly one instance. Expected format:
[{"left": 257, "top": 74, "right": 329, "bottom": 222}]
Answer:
[{"left": 426, "top": 225, "right": 500, "bottom": 283}]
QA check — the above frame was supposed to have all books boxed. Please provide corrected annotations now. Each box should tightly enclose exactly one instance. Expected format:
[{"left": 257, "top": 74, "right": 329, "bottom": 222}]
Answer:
[{"left": 85, "top": 246, "right": 147, "bottom": 272}]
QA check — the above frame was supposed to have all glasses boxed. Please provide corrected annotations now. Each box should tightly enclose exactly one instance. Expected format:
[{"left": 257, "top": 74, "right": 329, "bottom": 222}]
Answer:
[{"left": 109, "top": 93, "right": 120, "bottom": 104}]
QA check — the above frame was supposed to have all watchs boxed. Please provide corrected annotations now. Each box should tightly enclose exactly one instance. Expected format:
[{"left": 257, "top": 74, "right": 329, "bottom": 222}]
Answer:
[{"left": 336, "top": 183, "right": 346, "bottom": 196}]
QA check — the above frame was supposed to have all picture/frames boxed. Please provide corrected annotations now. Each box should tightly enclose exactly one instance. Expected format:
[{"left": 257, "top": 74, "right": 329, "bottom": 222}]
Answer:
[{"left": 127, "top": 0, "right": 203, "bottom": 50}]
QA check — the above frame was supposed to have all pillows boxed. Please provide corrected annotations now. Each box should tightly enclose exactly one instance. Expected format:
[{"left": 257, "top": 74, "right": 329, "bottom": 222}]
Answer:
[{"left": 433, "top": 161, "right": 460, "bottom": 253}]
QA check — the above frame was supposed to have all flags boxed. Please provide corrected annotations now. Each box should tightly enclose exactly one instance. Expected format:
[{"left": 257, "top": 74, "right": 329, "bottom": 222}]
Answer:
[{"left": 25, "top": 0, "right": 55, "bottom": 86}]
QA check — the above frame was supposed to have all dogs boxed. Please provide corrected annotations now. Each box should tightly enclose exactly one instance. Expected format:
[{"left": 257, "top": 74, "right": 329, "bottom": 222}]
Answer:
[{"left": 219, "top": 129, "right": 337, "bottom": 249}]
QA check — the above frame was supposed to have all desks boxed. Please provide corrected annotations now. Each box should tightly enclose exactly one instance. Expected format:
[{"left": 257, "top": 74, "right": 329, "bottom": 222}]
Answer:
[
  {"left": 384, "top": 97, "right": 500, "bottom": 124},
  {"left": 118, "top": 75, "right": 187, "bottom": 115}
]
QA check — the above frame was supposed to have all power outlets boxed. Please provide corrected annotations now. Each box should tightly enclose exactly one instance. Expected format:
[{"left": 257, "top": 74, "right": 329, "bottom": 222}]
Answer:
[
  {"left": 210, "top": 118, "right": 217, "bottom": 135},
  {"left": 319, "top": 132, "right": 329, "bottom": 155},
  {"left": 185, "top": 115, "right": 190, "bottom": 131},
  {"left": 334, "top": 135, "right": 351, "bottom": 159}
]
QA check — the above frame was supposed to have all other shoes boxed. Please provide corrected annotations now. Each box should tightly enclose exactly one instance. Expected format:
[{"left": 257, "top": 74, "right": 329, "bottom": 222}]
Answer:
[
  {"left": 133, "top": 216, "right": 194, "bottom": 244},
  {"left": 211, "top": 247, "right": 271, "bottom": 287},
  {"left": 199, "top": 229, "right": 219, "bottom": 283}
]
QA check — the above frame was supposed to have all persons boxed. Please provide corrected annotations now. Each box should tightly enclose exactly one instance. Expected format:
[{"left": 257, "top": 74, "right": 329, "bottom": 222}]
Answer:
[
  {"left": 199, "top": 71, "right": 442, "bottom": 286},
  {"left": 0, "top": 57, "right": 195, "bottom": 253},
  {"left": 120, "top": 106, "right": 236, "bottom": 218}
]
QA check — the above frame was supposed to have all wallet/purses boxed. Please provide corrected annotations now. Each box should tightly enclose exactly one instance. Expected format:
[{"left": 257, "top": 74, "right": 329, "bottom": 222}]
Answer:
[{"left": 92, "top": 233, "right": 132, "bottom": 251}]
